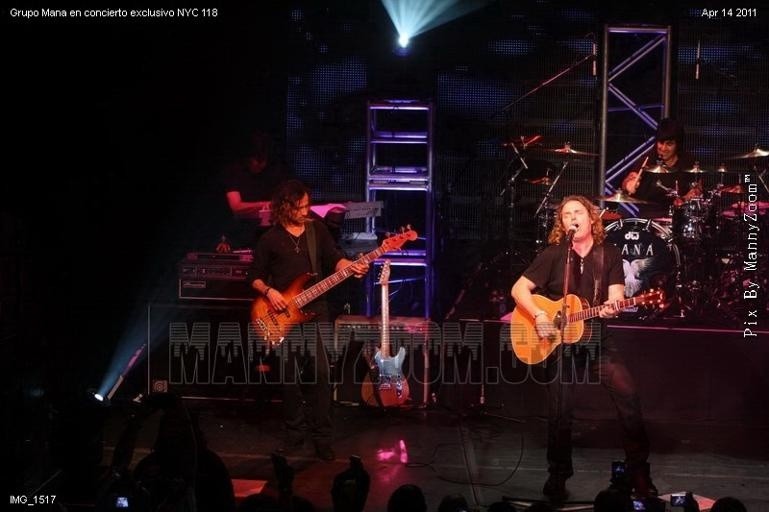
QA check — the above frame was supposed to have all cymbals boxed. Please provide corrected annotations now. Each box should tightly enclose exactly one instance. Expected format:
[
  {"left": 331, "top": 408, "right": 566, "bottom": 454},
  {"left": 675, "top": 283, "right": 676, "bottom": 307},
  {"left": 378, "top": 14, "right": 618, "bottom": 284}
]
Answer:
[
  {"left": 542, "top": 147, "right": 599, "bottom": 158},
  {"left": 727, "top": 149, "right": 769, "bottom": 160},
  {"left": 593, "top": 194, "right": 661, "bottom": 206},
  {"left": 718, "top": 185, "right": 752, "bottom": 196},
  {"left": 683, "top": 167, "right": 707, "bottom": 174},
  {"left": 521, "top": 176, "right": 576, "bottom": 187},
  {"left": 703, "top": 165, "right": 742, "bottom": 175}
]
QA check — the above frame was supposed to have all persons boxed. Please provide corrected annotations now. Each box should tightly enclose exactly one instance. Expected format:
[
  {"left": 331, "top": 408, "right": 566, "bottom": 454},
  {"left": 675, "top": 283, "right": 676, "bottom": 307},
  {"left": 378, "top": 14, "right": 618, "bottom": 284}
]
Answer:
[{"left": 125, "top": 116, "right": 749, "bottom": 512}]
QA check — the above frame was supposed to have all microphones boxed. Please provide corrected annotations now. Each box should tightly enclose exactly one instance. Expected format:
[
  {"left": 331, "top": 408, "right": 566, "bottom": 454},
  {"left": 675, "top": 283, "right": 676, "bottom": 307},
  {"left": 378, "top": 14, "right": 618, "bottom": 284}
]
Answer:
[
  {"left": 524, "top": 135, "right": 540, "bottom": 147},
  {"left": 592, "top": 42, "right": 597, "bottom": 76},
  {"left": 564, "top": 225, "right": 577, "bottom": 248},
  {"left": 695, "top": 40, "right": 701, "bottom": 80},
  {"left": 511, "top": 142, "right": 529, "bottom": 170}
]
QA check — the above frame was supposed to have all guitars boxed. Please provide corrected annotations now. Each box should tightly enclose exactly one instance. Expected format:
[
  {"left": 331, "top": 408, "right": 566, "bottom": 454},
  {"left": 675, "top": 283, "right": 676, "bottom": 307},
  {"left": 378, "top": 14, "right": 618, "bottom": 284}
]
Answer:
[
  {"left": 251, "top": 224, "right": 417, "bottom": 350},
  {"left": 360, "top": 257, "right": 410, "bottom": 407},
  {"left": 510, "top": 288, "right": 668, "bottom": 366}
]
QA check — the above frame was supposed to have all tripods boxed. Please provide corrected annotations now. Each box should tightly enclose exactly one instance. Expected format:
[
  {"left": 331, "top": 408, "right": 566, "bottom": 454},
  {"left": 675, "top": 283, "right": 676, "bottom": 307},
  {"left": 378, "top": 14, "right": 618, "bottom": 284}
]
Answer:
[
  {"left": 465, "top": 325, "right": 527, "bottom": 444},
  {"left": 501, "top": 249, "right": 595, "bottom": 504}
]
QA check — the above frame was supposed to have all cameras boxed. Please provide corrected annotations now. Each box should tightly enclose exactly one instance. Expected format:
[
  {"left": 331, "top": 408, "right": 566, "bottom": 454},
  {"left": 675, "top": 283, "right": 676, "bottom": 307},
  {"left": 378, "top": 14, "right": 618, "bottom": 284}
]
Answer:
[
  {"left": 671, "top": 496, "right": 688, "bottom": 507},
  {"left": 611, "top": 461, "right": 625, "bottom": 481},
  {"left": 632, "top": 499, "right": 648, "bottom": 512},
  {"left": 148, "top": 392, "right": 176, "bottom": 409},
  {"left": 113, "top": 495, "right": 131, "bottom": 509}
]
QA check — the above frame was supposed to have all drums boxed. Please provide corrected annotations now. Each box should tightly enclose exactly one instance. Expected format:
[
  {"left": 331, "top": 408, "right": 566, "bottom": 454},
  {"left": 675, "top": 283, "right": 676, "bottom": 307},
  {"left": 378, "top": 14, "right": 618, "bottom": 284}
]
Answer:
[
  {"left": 599, "top": 211, "right": 705, "bottom": 324},
  {"left": 710, "top": 209, "right": 753, "bottom": 264},
  {"left": 732, "top": 200, "right": 768, "bottom": 258}
]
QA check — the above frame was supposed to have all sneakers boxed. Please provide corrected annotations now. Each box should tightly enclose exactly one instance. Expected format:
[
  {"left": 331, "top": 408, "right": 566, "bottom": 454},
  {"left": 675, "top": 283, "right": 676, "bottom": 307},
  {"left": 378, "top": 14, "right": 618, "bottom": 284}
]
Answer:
[{"left": 543, "top": 475, "right": 566, "bottom": 496}]
